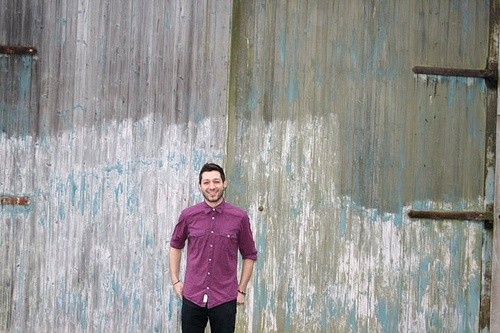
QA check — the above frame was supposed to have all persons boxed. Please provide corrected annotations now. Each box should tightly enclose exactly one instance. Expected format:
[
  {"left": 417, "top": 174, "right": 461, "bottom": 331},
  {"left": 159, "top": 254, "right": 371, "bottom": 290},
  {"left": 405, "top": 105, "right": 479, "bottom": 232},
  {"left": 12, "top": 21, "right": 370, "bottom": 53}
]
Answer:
[{"left": 168, "top": 163, "right": 257, "bottom": 333}]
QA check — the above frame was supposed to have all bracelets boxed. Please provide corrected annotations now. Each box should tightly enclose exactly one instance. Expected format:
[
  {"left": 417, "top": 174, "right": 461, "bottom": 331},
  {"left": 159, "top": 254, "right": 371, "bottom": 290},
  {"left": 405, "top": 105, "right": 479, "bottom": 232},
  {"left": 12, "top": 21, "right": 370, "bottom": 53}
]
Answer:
[
  {"left": 173, "top": 279, "right": 181, "bottom": 286},
  {"left": 237, "top": 289, "right": 247, "bottom": 296}
]
